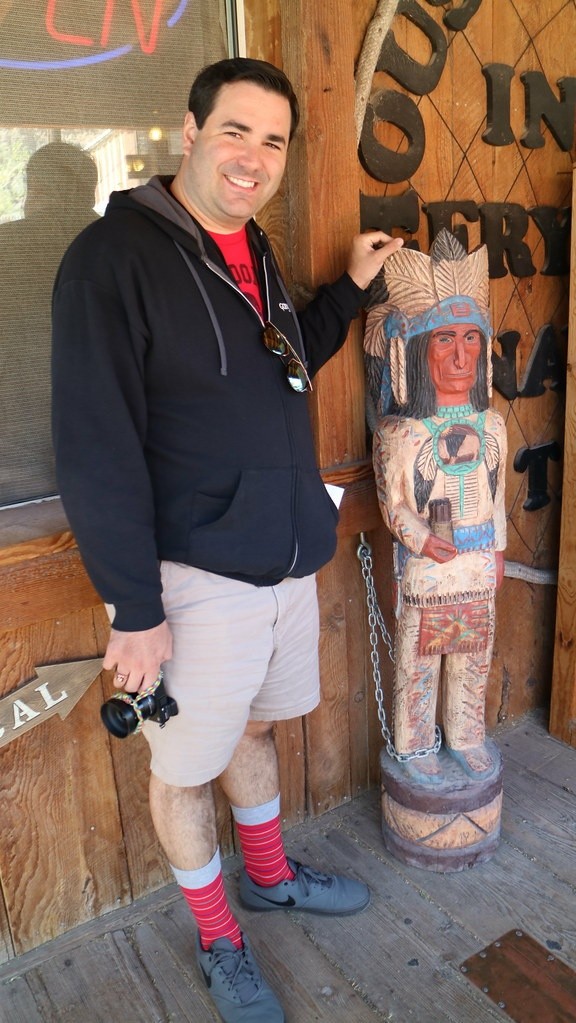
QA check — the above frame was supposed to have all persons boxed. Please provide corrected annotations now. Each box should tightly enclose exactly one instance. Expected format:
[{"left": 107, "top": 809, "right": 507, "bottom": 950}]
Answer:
[
  {"left": 0, "top": 142, "right": 101, "bottom": 512},
  {"left": 364, "top": 229, "right": 507, "bottom": 780},
  {"left": 50, "top": 60, "right": 404, "bottom": 1023}
]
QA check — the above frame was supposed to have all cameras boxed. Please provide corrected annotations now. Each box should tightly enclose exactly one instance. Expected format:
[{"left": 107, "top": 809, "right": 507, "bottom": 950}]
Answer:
[{"left": 100, "top": 676, "right": 179, "bottom": 739}]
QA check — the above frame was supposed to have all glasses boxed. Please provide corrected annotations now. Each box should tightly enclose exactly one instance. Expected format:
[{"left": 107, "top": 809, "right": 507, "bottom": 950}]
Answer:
[{"left": 262, "top": 319, "right": 313, "bottom": 393}]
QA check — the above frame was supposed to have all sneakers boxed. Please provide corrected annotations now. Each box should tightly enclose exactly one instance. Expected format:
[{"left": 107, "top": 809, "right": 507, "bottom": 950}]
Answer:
[
  {"left": 241, "top": 853, "right": 371, "bottom": 917},
  {"left": 196, "top": 924, "right": 284, "bottom": 1023}
]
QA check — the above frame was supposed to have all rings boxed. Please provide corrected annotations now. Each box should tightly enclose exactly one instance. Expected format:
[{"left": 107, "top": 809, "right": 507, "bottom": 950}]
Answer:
[{"left": 115, "top": 671, "right": 128, "bottom": 682}]
